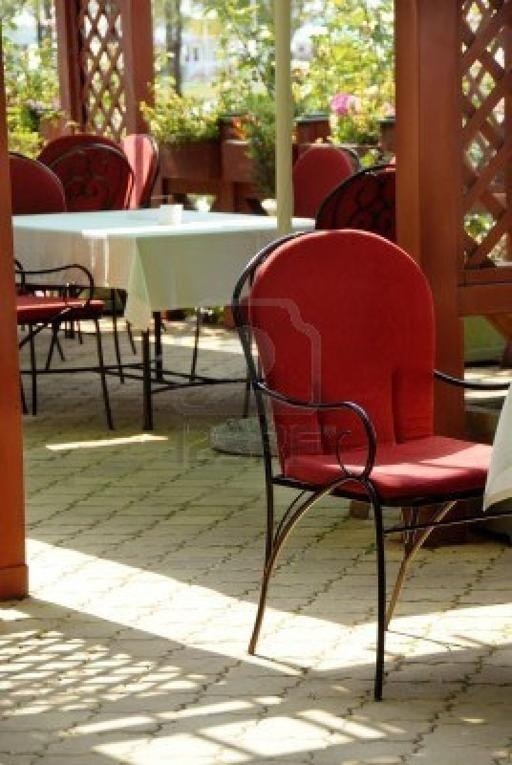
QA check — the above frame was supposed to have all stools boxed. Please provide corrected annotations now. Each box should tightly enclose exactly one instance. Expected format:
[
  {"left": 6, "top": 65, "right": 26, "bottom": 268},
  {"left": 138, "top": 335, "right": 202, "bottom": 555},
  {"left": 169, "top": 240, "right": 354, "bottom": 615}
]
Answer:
[{"left": 15, "top": 261, "right": 115, "bottom": 430}]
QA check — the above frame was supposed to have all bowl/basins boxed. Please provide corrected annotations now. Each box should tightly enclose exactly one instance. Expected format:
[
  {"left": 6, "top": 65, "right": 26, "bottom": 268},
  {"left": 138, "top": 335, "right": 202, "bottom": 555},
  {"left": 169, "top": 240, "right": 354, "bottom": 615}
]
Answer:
[
  {"left": 260, "top": 197, "right": 277, "bottom": 216},
  {"left": 188, "top": 192, "right": 218, "bottom": 214}
]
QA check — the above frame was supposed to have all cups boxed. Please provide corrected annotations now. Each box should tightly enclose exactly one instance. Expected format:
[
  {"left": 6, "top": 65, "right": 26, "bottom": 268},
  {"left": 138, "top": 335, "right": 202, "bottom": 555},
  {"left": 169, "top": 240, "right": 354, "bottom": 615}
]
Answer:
[{"left": 159, "top": 204, "right": 185, "bottom": 225}]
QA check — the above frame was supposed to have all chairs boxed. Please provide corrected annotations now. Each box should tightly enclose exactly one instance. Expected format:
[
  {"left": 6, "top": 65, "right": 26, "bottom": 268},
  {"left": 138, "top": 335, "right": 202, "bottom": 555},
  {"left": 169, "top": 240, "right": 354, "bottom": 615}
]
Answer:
[
  {"left": 318, "top": 162, "right": 396, "bottom": 242},
  {"left": 228, "top": 229, "right": 512, "bottom": 702},
  {"left": 7, "top": 132, "right": 363, "bottom": 210}
]
